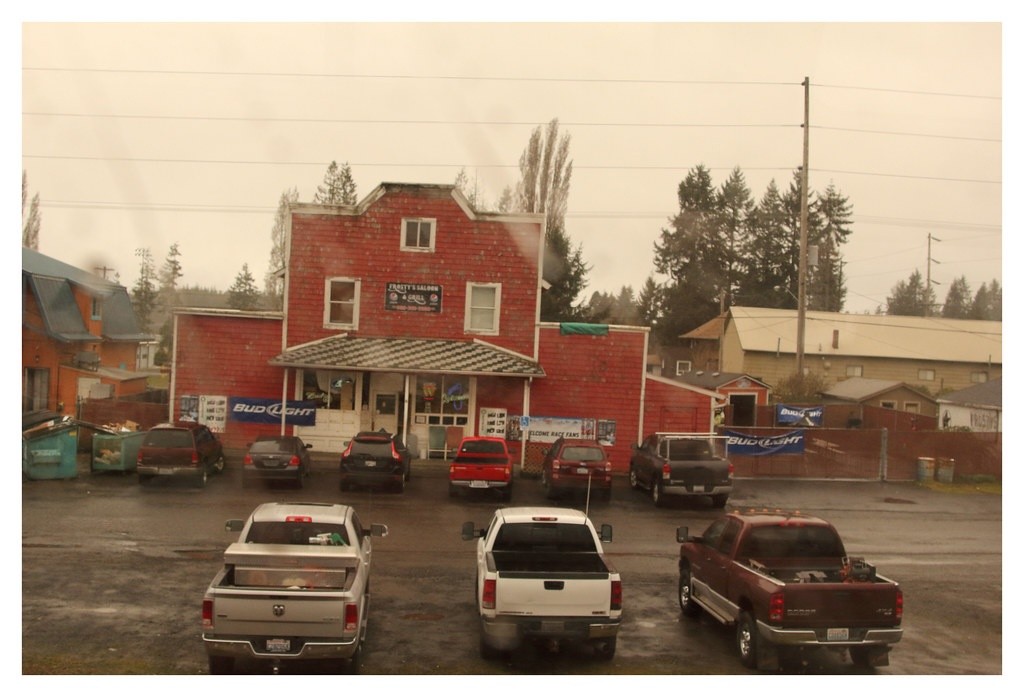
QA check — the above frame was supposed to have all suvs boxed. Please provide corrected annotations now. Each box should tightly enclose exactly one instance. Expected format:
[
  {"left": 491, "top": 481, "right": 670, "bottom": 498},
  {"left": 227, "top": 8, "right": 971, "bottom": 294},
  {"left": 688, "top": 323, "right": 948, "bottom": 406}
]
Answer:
[{"left": 339, "top": 431, "right": 412, "bottom": 494}]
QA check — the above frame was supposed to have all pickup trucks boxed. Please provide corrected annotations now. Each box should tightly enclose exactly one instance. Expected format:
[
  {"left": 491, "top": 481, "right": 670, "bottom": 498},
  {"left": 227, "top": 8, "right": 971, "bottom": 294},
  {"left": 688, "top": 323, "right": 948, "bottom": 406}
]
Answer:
[
  {"left": 676, "top": 509, "right": 905, "bottom": 668},
  {"left": 460, "top": 506, "right": 624, "bottom": 661},
  {"left": 449, "top": 436, "right": 513, "bottom": 501},
  {"left": 629, "top": 432, "right": 734, "bottom": 509},
  {"left": 201, "top": 500, "right": 389, "bottom": 676}
]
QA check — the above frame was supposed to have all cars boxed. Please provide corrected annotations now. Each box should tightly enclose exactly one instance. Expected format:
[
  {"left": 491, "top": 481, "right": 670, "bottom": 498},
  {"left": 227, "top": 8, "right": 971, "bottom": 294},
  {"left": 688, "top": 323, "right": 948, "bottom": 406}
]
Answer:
[
  {"left": 539, "top": 437, "right": 613, "bottom": 498},
  {"left": 243, "top": 434, "right": 313, "bottom": 490},
  {"left": 134, "top": 421, "right": 227, "bottom": 490}
]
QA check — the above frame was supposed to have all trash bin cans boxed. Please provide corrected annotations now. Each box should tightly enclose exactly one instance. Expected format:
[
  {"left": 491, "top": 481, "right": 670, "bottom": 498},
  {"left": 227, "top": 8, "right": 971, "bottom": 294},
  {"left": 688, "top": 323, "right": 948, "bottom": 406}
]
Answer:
[
  {"left": 917, "top": 457, "right": 955, "bottom": 484},
  {"left": 22, "top": 408, "right": 80, "bottom": 481},
  {"left": 90, "top": 428, "right": 149, "bottom": 472}
]
